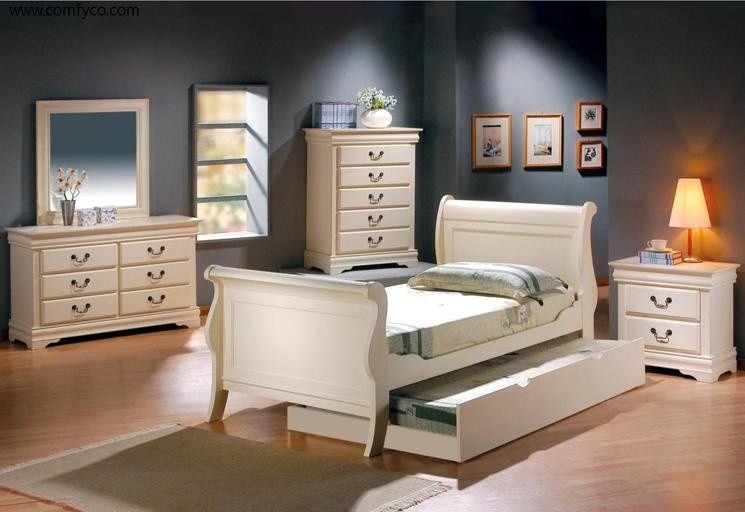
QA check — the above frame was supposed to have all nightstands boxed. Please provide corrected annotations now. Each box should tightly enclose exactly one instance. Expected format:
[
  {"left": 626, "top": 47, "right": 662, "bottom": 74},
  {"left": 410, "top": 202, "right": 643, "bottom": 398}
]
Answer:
[{"left": 608, "top": 256, "right": 740, "bottom": 382}]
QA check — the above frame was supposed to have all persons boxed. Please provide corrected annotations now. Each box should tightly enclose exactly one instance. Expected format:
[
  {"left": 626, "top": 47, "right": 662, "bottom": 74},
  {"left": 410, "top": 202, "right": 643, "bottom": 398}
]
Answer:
[{"left": 483, "top": 137, "right": 499, "bottom": 156}]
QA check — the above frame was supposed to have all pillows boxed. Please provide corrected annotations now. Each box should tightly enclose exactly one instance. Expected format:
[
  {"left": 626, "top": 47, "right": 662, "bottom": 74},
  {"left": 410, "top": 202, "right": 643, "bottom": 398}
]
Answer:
[{"left": 409, "top": 261, "right": 569, "bottom": 302}]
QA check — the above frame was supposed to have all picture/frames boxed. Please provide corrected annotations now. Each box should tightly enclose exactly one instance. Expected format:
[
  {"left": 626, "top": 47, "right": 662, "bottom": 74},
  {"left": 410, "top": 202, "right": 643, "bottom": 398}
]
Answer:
[
  {"left": 472, "top": 113, "right": 512, "bottom": 169},
  {"left": 576, "top": 140, "right": 604, "bottom": 171},
  {"left": 523, "top": 113, "right": 562, "bottom": 167},
  {"left": 577, "top": 101, "right": 603, "bottom": 131},
  {"left": 193, "top": 82, "right": 272, "bottom": 244}
]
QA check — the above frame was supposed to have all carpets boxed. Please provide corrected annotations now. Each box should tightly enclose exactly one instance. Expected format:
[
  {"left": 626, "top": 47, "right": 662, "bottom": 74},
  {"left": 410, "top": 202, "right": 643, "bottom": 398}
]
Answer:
[{"left": 0, "top": 422, "right": 452, "bottom": 512}]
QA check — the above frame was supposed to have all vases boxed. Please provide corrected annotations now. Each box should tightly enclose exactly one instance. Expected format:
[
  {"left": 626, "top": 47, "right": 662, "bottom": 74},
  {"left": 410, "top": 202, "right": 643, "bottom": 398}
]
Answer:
[
  {"left": 60, "top": 200, "right": 76, "bottom": 225},
  {"left": 359, "top": 108, "right": 392, "bottom": 128}
]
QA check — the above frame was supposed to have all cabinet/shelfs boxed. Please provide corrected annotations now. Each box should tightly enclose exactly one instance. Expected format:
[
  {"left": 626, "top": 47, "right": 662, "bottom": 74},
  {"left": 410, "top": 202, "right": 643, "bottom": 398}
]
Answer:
[
  {"left": 5, "top": 215, "right": 204, "bottom": 349},
  {"left": 204, "top": 194, "right": 645, "bottom": 464},
  {"left": 304, "top": 128, "right": 423, "bottom": 274}
]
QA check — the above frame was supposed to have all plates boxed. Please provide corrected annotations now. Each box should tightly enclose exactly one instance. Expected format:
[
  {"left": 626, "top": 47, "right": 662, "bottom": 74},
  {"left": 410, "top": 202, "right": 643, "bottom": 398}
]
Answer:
[{"left": 645, "top": 247, "right": 673, "bottom": 252}]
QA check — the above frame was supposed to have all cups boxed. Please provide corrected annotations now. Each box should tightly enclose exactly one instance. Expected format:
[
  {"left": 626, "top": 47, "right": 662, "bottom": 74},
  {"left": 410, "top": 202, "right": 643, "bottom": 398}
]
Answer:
[
  {"left": 53, "top": 198, "right": 63, "bottom": 210},
  {"left": 647, "top": 239, "right": 667, "bottom": 250}
]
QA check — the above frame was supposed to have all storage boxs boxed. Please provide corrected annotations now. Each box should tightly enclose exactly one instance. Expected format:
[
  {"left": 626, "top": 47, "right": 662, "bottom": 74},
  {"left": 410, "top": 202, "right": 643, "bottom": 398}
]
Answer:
[
  {"left": 77, "top": 209, "right": 96, "bottom": 227},
  {"left": 94, "top": 206, "right": 117, "bottom": 223}
]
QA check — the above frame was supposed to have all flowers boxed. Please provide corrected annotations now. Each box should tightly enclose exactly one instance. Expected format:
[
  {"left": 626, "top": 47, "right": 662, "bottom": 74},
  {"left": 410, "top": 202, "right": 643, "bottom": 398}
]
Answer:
[
  {"left": 54, "top": 168, "right": 88, "bottom": 200},
  {"left": 353, "top": 86, "right": 398, "bottom": 111}
]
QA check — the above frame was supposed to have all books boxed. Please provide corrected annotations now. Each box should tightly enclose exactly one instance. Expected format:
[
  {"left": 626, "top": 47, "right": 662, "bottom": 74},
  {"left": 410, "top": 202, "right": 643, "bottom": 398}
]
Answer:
[
  {"left": 639, "top": 250, "right": 683, "bottom": 266},
  {"left": 312, "top": 101, "right": 357, "bottom": 129}
]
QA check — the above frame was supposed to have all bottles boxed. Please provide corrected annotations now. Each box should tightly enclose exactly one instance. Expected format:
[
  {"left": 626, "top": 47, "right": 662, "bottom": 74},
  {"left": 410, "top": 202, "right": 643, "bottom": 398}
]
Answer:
[{"left": 62, "top": 200, "right": 76, "bottom": 225}]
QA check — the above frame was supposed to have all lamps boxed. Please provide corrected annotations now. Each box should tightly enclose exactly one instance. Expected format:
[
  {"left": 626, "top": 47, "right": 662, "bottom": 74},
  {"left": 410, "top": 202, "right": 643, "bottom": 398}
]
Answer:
[{"left": 668, "top": 178, "right": 711, "bottom": 262}]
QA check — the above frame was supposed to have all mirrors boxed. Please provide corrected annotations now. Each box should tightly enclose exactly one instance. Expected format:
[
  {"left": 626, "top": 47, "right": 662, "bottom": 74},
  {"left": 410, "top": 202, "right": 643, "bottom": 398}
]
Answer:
[{"left": 36, "top": 99, "right": 150, "bottom": 224}]
